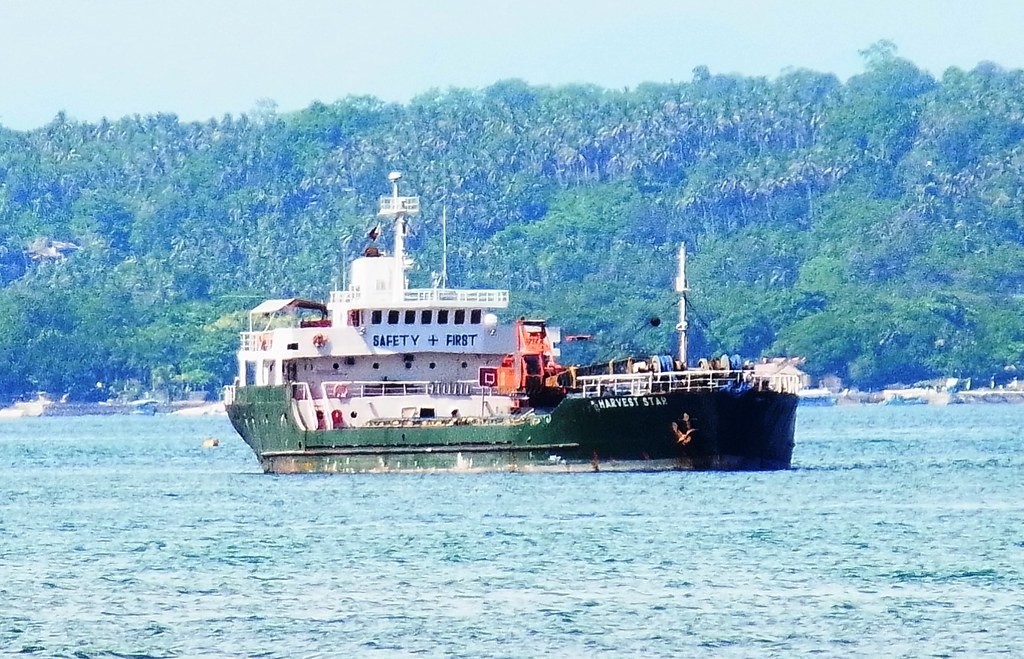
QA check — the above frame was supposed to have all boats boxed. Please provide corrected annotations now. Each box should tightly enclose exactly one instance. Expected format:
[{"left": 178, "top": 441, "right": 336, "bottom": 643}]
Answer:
[{"left": 222, "top": 172, "right": 800, "bottom": 471}]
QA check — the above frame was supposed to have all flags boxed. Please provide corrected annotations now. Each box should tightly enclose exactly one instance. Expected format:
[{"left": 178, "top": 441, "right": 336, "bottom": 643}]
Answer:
[{"left": 369, "top": 222, "right": 380, "bottom": 241}]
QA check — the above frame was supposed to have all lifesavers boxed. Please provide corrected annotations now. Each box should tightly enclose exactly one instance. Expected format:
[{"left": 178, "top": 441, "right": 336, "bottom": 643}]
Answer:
[{"left": 332, "top": 409, "right": 342, "bottom": 418}]
